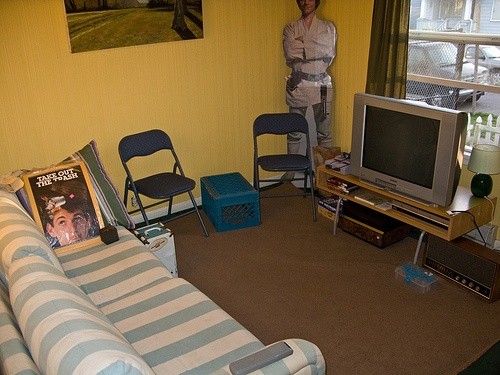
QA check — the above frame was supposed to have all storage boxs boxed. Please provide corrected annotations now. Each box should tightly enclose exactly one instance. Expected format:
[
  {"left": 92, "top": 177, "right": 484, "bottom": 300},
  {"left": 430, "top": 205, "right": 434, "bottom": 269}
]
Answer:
[
  {"left": 134, "top": 222, "right": 178, "bottom": 280},
  {"left": 318, "top": 196, "right": 413, "bottom": 249},
  {"left": 200, "top": 172, "right": 261, "bottom": 232},
  {"left": 394, "top": 262, "right": 439, "bottom": 291}
]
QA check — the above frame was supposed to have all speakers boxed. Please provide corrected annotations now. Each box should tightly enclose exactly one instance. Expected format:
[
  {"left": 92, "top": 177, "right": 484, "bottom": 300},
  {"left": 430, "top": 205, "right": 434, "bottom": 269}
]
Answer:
[{"left": 423, "top": 233, "right": 500, "bottom": 304}]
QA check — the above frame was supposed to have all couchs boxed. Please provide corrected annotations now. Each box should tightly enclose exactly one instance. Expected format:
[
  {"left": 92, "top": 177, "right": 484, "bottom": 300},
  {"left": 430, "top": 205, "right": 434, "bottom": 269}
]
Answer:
[{"left": 0, "top": 170, "right": 326, "bottom": 375}]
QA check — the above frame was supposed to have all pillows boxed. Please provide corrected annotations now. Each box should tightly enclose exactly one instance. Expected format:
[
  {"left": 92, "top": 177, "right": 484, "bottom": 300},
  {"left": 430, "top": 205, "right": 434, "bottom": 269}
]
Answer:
[{"left": 10, "top": 138, "right": 136, "bottom": 250}]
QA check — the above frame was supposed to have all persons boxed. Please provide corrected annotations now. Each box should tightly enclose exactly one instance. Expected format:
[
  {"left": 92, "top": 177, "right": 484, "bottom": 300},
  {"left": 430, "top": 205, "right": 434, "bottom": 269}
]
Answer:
[
  {"left": 45, "top": 193, "right": 92, "bottom": 247},
  {"left": 281, "top": 0, "right": 337, "bottom": 182}
]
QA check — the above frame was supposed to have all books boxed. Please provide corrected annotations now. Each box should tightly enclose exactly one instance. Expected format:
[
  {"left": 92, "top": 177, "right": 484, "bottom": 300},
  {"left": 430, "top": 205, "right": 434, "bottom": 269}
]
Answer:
[{"left": 319, "top": 176, "right": 396, "bottom": 211}]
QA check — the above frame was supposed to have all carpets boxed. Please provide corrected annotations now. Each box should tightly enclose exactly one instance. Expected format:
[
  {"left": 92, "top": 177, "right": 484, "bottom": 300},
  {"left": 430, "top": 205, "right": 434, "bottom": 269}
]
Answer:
[{"left": 130, "top": 183, "right": 500, "bottom": 375}]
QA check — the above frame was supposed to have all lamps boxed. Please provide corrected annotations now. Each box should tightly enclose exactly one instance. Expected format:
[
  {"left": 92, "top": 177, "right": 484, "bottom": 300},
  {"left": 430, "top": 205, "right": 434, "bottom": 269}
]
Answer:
[{"left": 467, "top": 144, "right": 500, "bottom": 198}]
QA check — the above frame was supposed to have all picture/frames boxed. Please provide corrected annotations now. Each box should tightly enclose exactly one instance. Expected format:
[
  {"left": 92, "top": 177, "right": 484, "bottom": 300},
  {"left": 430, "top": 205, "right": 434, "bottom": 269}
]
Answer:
[
  {"left": 22, "top": 161, "right": 106, "bottom": 254},
  {"left": 64, "top": 0, "right": 204, "bottom": 54}
]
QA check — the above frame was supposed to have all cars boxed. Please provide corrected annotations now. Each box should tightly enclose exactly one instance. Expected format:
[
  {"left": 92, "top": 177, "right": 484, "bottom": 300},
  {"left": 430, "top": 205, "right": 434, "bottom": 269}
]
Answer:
[
  {"left": 464, "top": 44, "right": 500, "bottom": 76},
  {"left": 409, "top": 40, "right": 487, "bottom": 102}
]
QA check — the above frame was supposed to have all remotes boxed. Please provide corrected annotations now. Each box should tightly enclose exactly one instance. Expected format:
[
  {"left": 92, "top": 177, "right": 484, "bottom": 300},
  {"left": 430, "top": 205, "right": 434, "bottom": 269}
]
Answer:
[{"left": 228, "top": 340, "right": 293, "bottom": 375}]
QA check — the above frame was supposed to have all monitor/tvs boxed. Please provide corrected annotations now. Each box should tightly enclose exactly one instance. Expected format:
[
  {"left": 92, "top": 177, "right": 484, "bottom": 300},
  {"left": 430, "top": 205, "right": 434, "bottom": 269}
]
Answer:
[{"left": 349, "top": 92, "right": 467, "bottom": 206}]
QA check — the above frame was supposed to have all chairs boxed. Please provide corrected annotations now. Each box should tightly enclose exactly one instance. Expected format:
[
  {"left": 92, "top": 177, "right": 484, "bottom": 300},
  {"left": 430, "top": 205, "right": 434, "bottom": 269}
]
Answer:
[
  {"left": 119, "top": 129, "right": 209, "bottom": 237},
  {"left": 253, "top": 113, "right": 316, "bottom": 222}
]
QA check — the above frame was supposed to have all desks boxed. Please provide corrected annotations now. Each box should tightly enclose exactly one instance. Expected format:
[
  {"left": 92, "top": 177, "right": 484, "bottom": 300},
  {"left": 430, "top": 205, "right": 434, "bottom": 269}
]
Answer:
[{"left": 317, "top": 161, "right": 497, "bottom": 265}]
